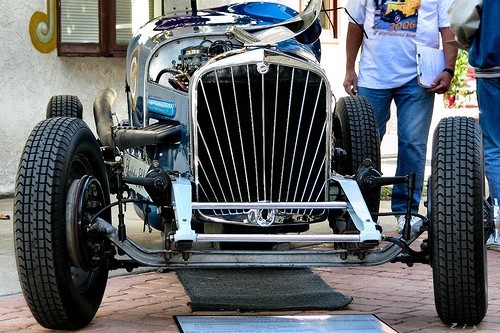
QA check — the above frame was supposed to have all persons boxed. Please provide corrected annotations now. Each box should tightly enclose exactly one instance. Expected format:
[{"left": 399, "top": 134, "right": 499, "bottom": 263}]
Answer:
[
  {"left": 342, "top": 0, "right": 458, "bottom": 236},
  {"left": 447, "top": 0, "right": 500, "bottom": 251}
]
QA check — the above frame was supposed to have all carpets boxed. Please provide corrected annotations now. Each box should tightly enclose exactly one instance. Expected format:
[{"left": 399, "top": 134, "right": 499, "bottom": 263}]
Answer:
[{"left": 174, "top": 266, "right": 353, "bottom": 311}]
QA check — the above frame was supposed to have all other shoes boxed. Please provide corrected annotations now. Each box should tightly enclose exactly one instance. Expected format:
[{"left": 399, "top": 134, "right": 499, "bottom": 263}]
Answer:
[{"left": 486, "top": 233, "right": 500, "bottom": 251}]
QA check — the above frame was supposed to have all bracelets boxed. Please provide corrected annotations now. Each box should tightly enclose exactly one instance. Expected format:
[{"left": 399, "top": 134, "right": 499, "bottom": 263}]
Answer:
[{"left": 443, "top": 68, "right": 454, "bottom": 78}]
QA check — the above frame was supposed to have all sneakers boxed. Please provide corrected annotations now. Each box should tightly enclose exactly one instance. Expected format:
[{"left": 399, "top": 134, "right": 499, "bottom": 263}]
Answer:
[{"left": 398, "top": 212, "right": 415, "bottom": 232}]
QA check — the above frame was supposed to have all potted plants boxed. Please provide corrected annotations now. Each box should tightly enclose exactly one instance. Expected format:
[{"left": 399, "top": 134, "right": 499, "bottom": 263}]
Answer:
[{"left": 444, "top": 52, "right": 468, "bottom": 106}]
